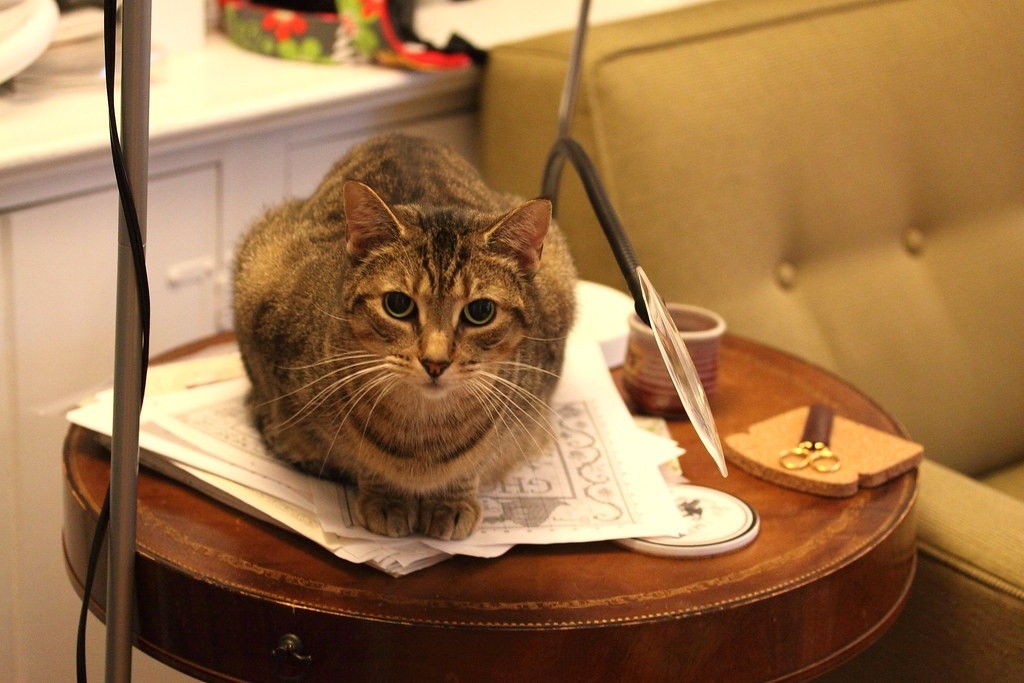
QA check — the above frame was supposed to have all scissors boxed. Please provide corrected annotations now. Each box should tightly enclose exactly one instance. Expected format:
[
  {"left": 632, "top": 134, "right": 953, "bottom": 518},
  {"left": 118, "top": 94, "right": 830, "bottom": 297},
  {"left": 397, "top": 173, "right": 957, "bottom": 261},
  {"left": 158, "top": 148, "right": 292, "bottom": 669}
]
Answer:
[{"left": 778, "top": 403, "right": 841, "bottom": 473}]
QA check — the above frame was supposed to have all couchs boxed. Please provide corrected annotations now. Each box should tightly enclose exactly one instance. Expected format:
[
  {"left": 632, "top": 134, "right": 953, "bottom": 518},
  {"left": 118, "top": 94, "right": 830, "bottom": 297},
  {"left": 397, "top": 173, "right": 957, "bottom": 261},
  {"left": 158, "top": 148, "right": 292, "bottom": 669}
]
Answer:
[{"left": 482, "top": 0, "right": 1023, "bottom": 682}]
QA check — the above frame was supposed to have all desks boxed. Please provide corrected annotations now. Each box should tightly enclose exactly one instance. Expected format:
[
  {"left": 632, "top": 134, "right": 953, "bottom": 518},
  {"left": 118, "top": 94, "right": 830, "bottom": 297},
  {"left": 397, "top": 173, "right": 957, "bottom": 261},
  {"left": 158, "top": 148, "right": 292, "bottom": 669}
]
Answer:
[{"left": 61, "top": 321, "right": 917, "bottom": 683}]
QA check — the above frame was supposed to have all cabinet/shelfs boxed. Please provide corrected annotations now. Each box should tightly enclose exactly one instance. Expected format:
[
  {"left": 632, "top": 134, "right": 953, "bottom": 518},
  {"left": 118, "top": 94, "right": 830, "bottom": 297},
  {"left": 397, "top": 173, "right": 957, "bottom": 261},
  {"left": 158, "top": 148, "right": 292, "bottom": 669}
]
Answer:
[{"left": 0, "top": 1, "right": 693, "bottom": 683}]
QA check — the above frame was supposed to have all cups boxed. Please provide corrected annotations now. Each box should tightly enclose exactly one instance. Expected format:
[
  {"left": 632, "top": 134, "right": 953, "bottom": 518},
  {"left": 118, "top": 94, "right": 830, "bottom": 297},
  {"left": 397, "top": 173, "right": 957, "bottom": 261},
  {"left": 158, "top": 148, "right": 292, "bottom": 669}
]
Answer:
[{"left": 622, "top": 303, "right": 726, "bottom": 416}]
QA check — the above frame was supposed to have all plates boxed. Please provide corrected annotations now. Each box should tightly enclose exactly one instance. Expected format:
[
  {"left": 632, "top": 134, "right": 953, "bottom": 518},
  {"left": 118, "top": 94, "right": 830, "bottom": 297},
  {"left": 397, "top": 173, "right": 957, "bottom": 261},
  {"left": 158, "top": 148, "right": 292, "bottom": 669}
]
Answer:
[
  {"left": 0, "top": 0, "right": 61, "bottom": 84},
  {"left": 616, "top": 482, "right": 760, "bottom": 556}
]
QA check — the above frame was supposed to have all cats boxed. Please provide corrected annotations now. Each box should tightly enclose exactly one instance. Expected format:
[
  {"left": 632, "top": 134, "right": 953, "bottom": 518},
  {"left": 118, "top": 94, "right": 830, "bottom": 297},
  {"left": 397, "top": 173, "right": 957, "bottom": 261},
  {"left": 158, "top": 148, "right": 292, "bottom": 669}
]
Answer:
[{"left": 233, "top": 132, "right": 577, "bottom": 540}]
